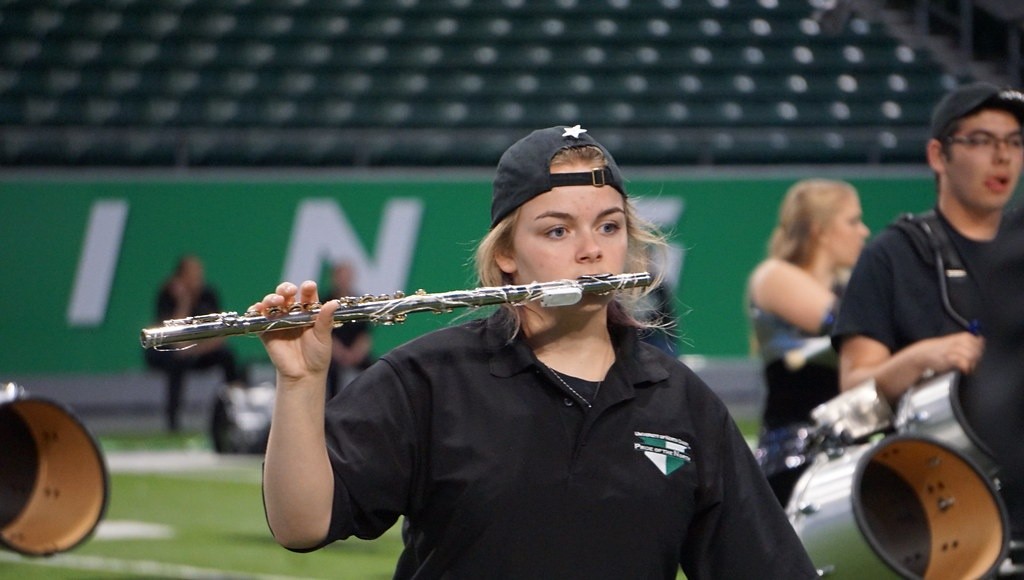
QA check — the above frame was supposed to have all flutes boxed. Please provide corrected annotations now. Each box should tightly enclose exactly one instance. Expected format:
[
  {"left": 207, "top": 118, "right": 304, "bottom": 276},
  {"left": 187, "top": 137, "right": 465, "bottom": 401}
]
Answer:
[{"left": 140, "top": 272, "right": 652, "bottom": 355}]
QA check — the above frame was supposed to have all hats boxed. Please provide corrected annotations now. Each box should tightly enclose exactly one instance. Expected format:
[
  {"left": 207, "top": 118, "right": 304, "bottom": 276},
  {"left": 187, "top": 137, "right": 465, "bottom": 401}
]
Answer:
[
  {"left": 931, "top": 81, "right": 1024, "bottom": 139},
  {"left": 491, "top": 126, "right": 628, "bottom": 229}
]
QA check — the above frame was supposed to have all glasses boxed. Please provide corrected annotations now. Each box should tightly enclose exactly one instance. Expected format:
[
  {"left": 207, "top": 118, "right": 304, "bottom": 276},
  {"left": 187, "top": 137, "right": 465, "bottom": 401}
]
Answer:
[{"left": 944, "top": 124, "right": 1024, "bottom": 156}]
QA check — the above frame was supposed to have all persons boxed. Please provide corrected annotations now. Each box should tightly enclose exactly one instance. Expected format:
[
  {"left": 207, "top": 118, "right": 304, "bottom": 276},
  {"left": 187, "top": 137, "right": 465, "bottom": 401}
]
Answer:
[
  {"left": 153, "top": 254, "right": 238, "bottom": 433},
  {"left": 249, "top": 126, "right": 821, "bottom": 580},
  {"left": 321, "top": 264, "right": 374, "bottom": 399},
  {"left": 833, "top": 80, "right": 1024, "bottom": 580},
  {"left": 749, "top": 179, "right": 873, "bottom": 508},
  {"left": 637, "top": 270, "right": 680, "bottom": 358}
]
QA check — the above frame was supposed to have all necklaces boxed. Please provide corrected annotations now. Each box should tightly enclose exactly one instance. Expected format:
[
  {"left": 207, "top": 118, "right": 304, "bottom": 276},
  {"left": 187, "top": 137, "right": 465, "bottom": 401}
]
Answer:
[{"left": 543, "top": 343, "right": 611, "bottom": 408}]
